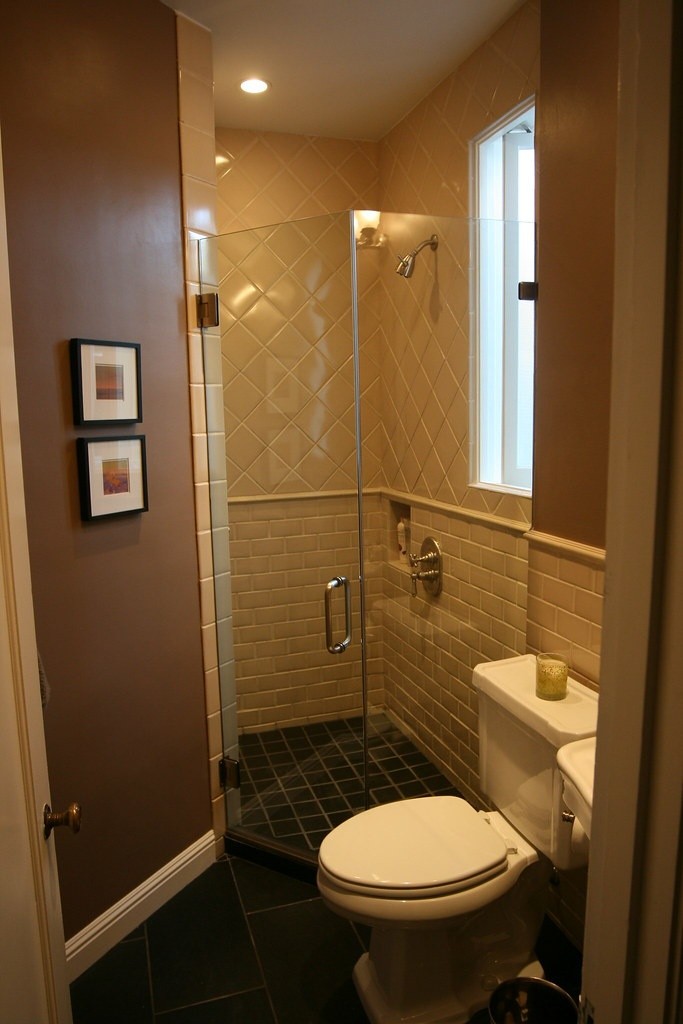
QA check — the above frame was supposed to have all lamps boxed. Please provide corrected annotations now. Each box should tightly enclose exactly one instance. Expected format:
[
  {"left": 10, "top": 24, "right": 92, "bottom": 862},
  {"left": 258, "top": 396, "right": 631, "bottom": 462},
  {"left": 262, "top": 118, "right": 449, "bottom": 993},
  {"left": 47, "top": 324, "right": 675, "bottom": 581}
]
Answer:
[{"left": 353, "top": 210, "right": 388, "bottom": 250}]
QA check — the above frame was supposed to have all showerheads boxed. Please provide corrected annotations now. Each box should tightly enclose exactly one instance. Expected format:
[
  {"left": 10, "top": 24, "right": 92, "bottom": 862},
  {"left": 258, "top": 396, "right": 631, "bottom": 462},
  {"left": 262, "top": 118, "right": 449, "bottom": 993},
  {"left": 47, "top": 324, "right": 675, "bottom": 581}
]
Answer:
[{"left": 393, "top": 248, "right": 419, "bottom": 279}]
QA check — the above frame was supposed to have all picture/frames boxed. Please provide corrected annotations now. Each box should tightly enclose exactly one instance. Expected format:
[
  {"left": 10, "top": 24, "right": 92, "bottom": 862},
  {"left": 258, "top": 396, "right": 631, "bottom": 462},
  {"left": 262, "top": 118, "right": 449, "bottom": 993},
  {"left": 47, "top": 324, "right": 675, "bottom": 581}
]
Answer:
[
  {"left": 68, "top": 338, "right": 143, "bottom": 426},
  {"left": 76, "top": 436, "right": 149, "bottom": 520}
]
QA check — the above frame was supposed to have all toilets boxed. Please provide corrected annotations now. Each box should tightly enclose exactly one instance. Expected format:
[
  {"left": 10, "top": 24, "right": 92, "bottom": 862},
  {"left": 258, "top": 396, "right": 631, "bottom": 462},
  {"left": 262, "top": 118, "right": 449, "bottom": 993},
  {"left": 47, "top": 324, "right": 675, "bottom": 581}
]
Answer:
[{"left": 315, "top": 652, "right": 600, "bottom": 1024}]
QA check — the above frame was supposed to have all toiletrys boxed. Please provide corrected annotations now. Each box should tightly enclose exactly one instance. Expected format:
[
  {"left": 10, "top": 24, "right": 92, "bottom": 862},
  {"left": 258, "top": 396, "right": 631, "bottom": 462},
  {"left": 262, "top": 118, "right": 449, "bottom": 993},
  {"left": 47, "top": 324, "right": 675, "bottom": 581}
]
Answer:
[{"left": 396, "top": 516, "right": 413, "bottom": 568}]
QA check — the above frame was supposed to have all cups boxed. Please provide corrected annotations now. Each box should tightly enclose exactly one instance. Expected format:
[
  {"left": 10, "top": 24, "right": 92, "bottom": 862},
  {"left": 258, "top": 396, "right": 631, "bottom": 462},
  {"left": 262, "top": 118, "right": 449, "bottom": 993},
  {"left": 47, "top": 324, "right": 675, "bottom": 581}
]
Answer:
[{"left": 536, "top": 652, "right": 568, "bottom": 701}]
